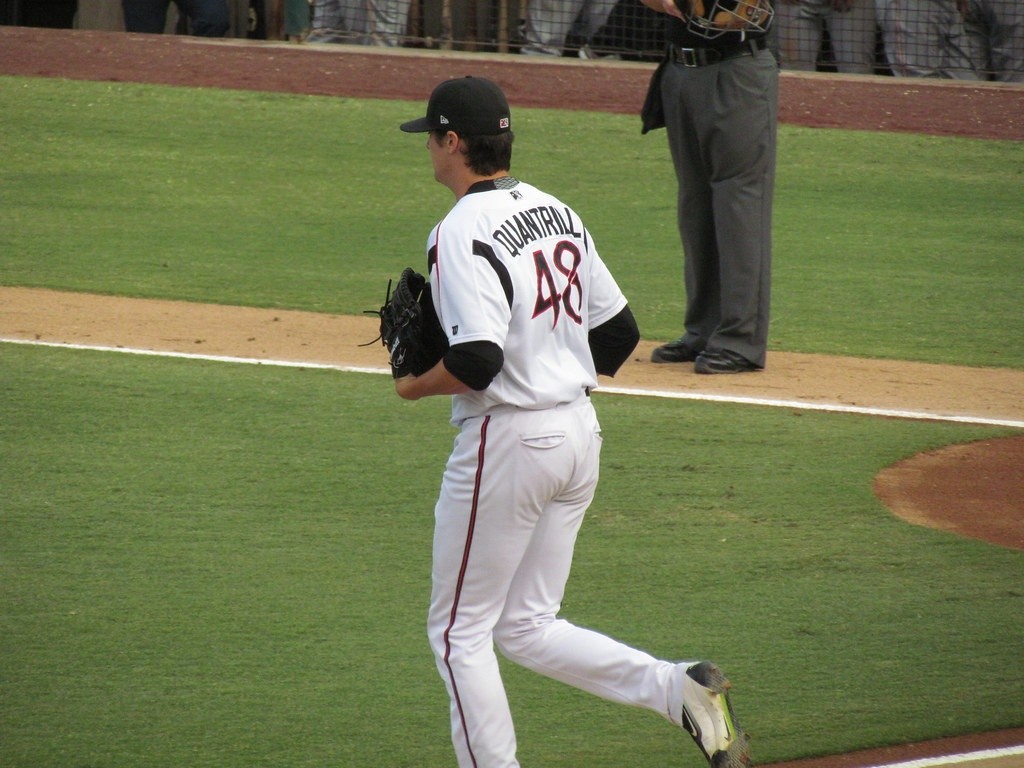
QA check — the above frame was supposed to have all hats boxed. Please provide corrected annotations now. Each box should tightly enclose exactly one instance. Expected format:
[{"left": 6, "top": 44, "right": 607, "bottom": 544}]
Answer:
[{"left": 400, "top": 76, "right": 510, "bottom": 135}]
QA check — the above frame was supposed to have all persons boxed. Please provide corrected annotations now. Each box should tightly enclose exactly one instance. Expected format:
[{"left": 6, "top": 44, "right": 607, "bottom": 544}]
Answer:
[
  {"left": 394, "top": 76, "right": 756, "bottom": 768},
  {"left": 641, "top": 0, "right": 778, "bottom": 374},
  {"left": 121, "top": 0, "right": 1024, "bottom": 83}
]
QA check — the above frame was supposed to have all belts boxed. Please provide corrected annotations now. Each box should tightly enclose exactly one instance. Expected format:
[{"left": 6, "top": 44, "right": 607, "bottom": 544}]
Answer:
[{"left": 668, "top": 37, "right": 767, "bottom": 67}]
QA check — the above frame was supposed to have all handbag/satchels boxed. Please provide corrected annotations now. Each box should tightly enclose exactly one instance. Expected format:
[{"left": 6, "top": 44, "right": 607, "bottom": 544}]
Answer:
[{"left": 641, "top": 62, "right": 666, "bottom": 135}]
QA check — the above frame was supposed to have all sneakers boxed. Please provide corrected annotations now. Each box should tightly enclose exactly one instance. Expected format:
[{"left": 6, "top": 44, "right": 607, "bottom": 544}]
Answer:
[{"left": 681, "top": 660, "right": 754, "bottom": 768}]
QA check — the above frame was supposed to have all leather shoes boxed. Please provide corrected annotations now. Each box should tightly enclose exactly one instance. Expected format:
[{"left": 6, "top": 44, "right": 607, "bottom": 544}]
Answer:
[
  {"left": 695, "top": 346, "right": 756, "bottom": 374},
  {"left": 651, "top": 339, "right": 697, "bottom": 363}
]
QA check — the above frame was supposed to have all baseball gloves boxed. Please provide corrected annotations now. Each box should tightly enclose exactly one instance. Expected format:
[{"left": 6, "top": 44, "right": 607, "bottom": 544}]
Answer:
[{"left": 379, "top": 264, "right": 451, "bottom": 378}]
